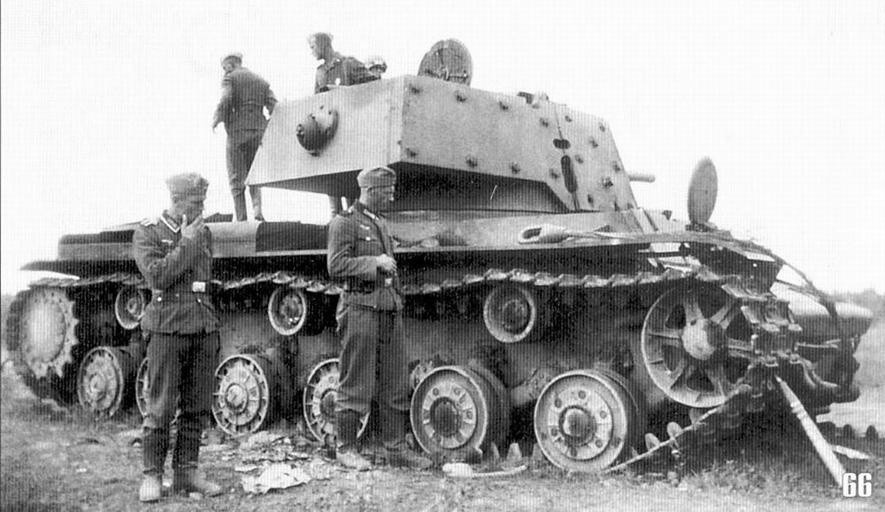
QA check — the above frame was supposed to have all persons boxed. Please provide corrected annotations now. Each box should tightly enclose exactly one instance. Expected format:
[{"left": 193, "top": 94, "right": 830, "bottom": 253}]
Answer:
[
  {"left": 309, "top": 31, "right": 373, "bottom": 220},
  {"left": 326, "top": 164, "right": 433, "bottom": 474},
  {"left": 131, "top": 170, "right": 224, "bottom": 503},
  {"left": 211, "top": 51, "right": 280, "bottom": 221}
]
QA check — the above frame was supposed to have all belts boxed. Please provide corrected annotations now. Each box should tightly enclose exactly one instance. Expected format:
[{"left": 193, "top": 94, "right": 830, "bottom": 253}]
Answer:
[
  {"left": 372, "top": 276, "right": 396, "bottom": 289},
  {"left": 167, "top": 282, "right": 216, "bottom": 295}
]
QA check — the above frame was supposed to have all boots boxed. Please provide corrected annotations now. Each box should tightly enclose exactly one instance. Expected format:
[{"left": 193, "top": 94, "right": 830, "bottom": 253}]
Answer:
[
  {"left": 171, "top": 428, "right": 221, "bottom": 497},
  {"left": 138, "top": 429, "right": 170, "bottom": 503},
  {"left": 335, "top": 410, "right": 371, "bottom": 472},
  {"left": 378, "top": 409, "right": 435, "bottom": 470}
]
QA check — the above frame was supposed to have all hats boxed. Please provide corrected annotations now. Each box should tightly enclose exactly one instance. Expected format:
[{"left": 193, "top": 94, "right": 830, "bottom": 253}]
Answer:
[
  {"left": 357, "top": 165, "right": 398, "bottom": 189},
  {"left": 220, "top": 50, "right": 244, "bottom": 62},
  {"left": 165, "top": 172, "right": 209, "bottom": 198}
]
